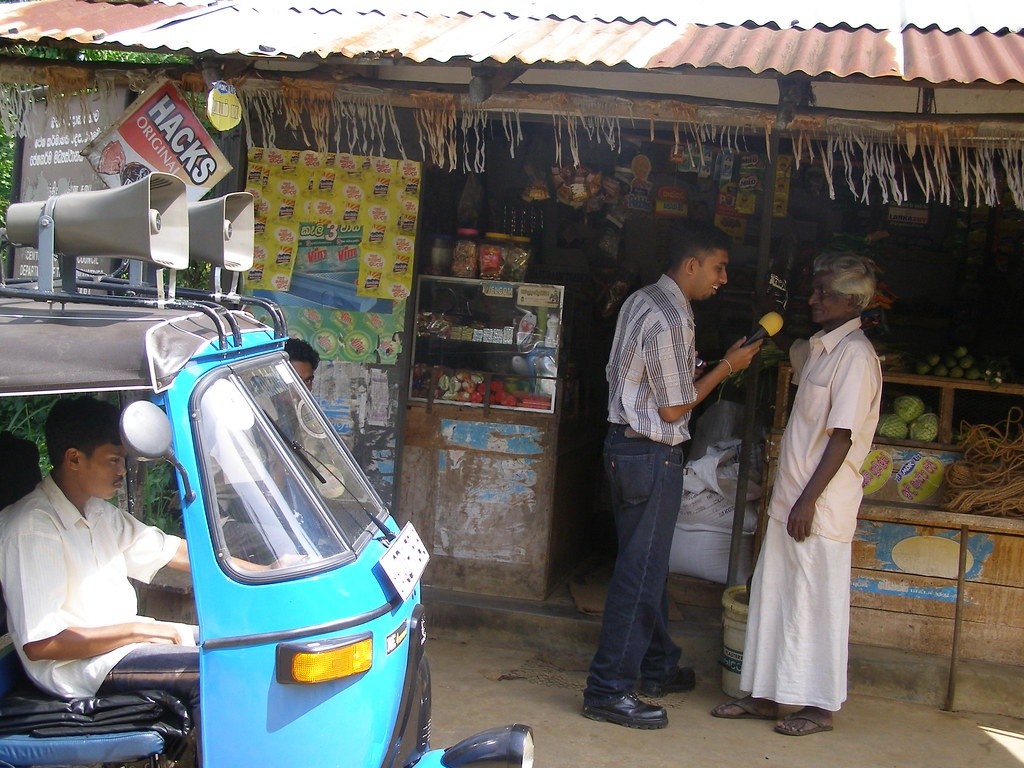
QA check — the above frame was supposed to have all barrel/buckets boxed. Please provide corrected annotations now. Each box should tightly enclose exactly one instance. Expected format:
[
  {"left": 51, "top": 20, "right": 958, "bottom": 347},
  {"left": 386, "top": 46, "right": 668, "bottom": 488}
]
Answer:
[
  {"left": 720, "top": 585, "right": 752, "bottom": 699},
  {"left": 686, "top": 398, "right": 737, "bottom": 460}
]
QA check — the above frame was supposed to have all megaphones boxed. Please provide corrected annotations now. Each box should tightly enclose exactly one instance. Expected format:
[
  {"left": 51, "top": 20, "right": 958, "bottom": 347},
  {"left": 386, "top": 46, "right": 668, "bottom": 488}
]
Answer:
[{"left": 6, "top": 172, "right": 254, "bottom": 271}]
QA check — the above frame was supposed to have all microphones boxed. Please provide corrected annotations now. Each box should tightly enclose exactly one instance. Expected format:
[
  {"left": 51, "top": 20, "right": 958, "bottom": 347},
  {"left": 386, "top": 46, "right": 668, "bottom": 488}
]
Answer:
[{"left": 740, "top": 312, "right": 783, "bottom": 350}]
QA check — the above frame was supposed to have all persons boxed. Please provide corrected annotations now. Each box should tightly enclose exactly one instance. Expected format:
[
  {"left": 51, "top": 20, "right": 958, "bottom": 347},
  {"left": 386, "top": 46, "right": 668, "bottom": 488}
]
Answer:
[
  {"left": 0, "top": 395, "right": 314, "bottom": 768},
  {"left": 581, "top": 224, "right": 765, "bottom": 732},
  {"left": 202, "top": 337, "right": 323, "bottom": 566},
  {"left": 711, "top": 250, "right": 883, "bottom": 735}
]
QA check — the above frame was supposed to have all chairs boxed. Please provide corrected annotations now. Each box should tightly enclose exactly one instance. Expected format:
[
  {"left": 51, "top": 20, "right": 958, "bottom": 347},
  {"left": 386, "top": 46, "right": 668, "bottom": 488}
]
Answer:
[{"left": 0, "top": 432, "right": 204, "bottom": 768}]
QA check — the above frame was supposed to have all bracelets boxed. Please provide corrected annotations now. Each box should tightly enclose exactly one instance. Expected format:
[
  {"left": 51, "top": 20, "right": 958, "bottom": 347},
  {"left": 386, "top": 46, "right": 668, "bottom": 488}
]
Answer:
[{"left": 718, "top": 358, "right": 733, "bottom": 376}]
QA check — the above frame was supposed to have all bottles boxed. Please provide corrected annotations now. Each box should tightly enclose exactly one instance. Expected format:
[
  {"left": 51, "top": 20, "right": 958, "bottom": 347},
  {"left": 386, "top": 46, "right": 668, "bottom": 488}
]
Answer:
[{"left": 544, "top": 313, "right": 559, "bottom": 348}]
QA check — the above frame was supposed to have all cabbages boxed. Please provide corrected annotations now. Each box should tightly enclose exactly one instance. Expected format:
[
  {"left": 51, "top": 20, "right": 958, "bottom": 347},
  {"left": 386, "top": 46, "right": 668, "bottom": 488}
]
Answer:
[{"left": 877, "top": 394, "right": 939, "bottom": 441}]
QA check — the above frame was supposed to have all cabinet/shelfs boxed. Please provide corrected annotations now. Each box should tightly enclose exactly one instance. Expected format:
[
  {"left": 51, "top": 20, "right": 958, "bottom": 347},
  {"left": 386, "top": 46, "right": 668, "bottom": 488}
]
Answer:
[
  {"left": 754, "top": 361, "right": 1024, "bottom": 540},
  {"left": 409, "top": 273, "right": 564, "bottom": 411}
]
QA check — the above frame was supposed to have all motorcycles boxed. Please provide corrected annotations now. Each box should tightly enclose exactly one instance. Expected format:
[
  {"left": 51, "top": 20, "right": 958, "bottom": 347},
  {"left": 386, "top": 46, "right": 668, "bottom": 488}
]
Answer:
[{"left": 0, "top": 169, "right": 535, "bottom": 768}]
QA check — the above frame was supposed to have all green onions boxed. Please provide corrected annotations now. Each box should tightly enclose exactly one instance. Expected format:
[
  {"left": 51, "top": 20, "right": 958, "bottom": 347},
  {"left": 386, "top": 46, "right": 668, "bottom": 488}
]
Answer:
[{"left": 718, "top": 342, "right": 790, "bottom": 404}]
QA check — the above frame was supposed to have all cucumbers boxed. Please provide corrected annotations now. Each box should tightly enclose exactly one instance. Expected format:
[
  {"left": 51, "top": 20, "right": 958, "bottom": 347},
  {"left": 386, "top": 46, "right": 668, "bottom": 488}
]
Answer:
[{"left": 915, "top": 346, "right": 987, "bottom": 381}]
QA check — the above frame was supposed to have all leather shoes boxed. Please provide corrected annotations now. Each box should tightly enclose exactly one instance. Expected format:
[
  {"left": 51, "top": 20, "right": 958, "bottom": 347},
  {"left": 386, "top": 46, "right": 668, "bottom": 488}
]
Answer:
[
  {"left": 638, "top": 667, "right": 696, "bottom": 698},
  {"left": 583, "top": 691, "right": 668, "bottom": 729}
]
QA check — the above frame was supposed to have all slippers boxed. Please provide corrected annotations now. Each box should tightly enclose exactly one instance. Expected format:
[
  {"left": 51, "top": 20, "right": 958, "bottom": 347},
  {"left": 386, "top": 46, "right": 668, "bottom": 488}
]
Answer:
[
  {"left": 711, "top": 698, "right": 778, "bottom": 720},
  {"left": 775, "top": 713, "right": 833, "bottom": 735}
]
